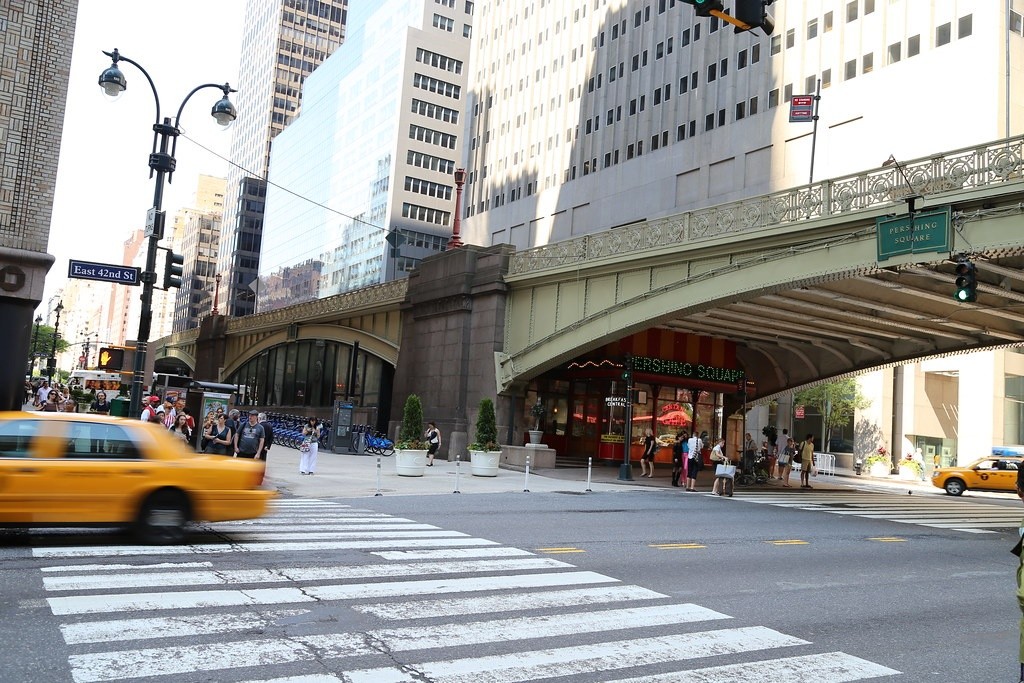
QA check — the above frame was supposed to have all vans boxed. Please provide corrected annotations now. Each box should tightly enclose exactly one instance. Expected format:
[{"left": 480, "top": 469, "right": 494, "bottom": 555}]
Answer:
[{"left": 65, "top": 370, "right": 192, "bottom": 415}]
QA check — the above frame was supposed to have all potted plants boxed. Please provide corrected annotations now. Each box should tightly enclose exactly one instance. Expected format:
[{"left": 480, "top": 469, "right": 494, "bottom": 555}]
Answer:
[
  {"left": 898, "top": 459, "right": 921, "bottom": 481},
  {"left": 866, "top": 456, "right": 893, "bottom": 477},
  {"left": 467, "top": 398, "right": 503, "bottom": 476},
  {"left": 529, "top": 403, "right": 545, "bottom": 444},
  {"left": 394, "top": 394, "right": 430, "bottom": 477}
]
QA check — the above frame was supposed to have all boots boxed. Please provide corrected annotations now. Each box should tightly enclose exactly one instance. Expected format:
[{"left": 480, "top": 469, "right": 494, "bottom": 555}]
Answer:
[
  {"left": 674, "top": 472, "right": 680, "bottom": 487},
  {"left": 672, "top": 472, "right": 675, "bottom": 486}
]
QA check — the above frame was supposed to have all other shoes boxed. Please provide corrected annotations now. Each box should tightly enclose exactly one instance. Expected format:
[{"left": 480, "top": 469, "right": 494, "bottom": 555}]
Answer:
[
  {"left": 686, "top": 488, "right": 697, "bottom": 492},
  {"left": 805, "top": 484, "right": 812, "bottom": 488},
  {"left": 301, "top": 472, "right": 304, "bottom": 475},
  {"left": 640, "top": 473, "right": 646, "bottom": 477},
  {"left": 801, "top": 485, "right": 805, "bottom": 488},
  {"left": 783, "top": 483, "right": 792, "bottom": 487},
  {"left": 648, "top": 474, "right": 654, "bottom": 478},
  {"left": 427, "top": 464, "right": 433, "bottom": 466},
  {"left": 778, "top": 477, "right": 783, "bottom": 480},
  {"left": 310, "top": 472, "right": 313, "bottom": 475}
]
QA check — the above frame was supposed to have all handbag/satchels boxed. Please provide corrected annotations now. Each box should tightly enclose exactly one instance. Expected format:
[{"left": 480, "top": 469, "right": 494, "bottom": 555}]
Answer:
[
  {"left": 715, "top": 460, "right": 736, "bottom": 480},
  {"left": 778, "top": 451, "right": 789, "bottom": 464},
  {"left": 746, "top": 450, "right": 754, "bottom": 460},
  {"left": 300, "top": 441, "right": 310, "bottom": 452},
  {"left": 694, "top": 453, "right": 698, "bottom": 463},
  {"left": 793, "top": 454, "right": 802, "bottom": 463},
  {"left": 811, "top": 466, "right": 818, "bottom": 476}
]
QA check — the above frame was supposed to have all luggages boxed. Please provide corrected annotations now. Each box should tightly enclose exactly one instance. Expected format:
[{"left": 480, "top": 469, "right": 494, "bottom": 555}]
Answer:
[{"left": 719, "top": 458, "right": 733, "bottom": 497}]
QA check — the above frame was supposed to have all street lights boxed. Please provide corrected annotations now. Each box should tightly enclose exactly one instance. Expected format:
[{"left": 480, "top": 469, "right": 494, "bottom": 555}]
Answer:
[
  {"left": 28, "top": 313, "right": 43, "bottom": 382},
  {"left": 48, "top": 300, "right": 64, "bottom": 387},
  {"left": 95, "top": 47, "right": 239, "bottom": 421}
]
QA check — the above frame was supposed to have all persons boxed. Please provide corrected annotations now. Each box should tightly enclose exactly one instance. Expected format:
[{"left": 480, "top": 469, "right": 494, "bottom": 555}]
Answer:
[
  {"left": 672, "top": 431, "right": 704, "bottom": 491},
  {"left": 711, "top": 439, "right": 728, "bottom": 495},
  {"left": 423, "top": 421, "right": 441, "bottom": 466},
  {"left": 140, "top": 396, "right": 195, "bottom": 444},
  {"left": 202, "top": 409, "right": 273, "bottom": 461},
  {"left": 746, "top": 433, "right": 758, "bottom": 451},
  {"left": 22, "top": 379, "right": 71, "bottom": 411},
  {"left": 640, "top": 429, "right": 656, "bottom": 478},
  {"left": 300, "top": 416, "right": 320, "bottom": 475},
  {"left": 798, "top": 434, "right": 815, "bottom": 489},
  {"left": 761, "top": 428, "right": 796, "bottom": 487},
  {"left": 90, "top": 385, "right": 111, "bottom": 413},
  {"left": 991, "top": 463, "right": 999, "bottom": 470}
]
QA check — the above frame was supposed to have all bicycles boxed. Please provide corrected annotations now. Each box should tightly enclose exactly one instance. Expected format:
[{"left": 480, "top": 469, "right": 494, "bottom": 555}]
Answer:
[
  {"left": 236, "top": 409, "right": 333, "bottom": 451},
  {"left": 350, "top": 423, "right": 396, "bottom": 457},
  {"left": 731, "top": 450, "right": 771, "bottom": 487}
]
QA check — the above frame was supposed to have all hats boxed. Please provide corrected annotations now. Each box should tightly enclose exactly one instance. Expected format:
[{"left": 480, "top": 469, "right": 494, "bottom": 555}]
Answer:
[
  {"left": 249, "top": 410, "right": 258, "bottom": 415},
  {"left": 167, "top": 396, "right": 174, "bottom": 403},
  {"left": 150, "top": 396, "right": 160, "bottom": 401}
]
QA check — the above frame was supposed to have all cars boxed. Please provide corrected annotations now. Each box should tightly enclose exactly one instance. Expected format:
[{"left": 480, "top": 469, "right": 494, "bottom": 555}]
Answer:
[
  {"left": 0, "top": 409, "right": 279, "bottom": 546},
  {"left": 931, "top": 446, "right": 1024, "bottom": 496}
]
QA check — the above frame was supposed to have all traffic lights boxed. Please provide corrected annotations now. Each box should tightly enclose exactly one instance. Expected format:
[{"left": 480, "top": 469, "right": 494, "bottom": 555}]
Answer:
[
  {"left": 97, "top": 346, "right": 125, "bottom": 371},
  {"left": 953, "top": 259, "right": 979, "bottom": 303},
  {"left": 621, "top": 359, "right": 632, "bottom": 380},
  {"left": 163, "top": 248, "right": 184, "bottom": 290},
  {"left": 635, "top": 390, "right": 648, "bottom": 405}
]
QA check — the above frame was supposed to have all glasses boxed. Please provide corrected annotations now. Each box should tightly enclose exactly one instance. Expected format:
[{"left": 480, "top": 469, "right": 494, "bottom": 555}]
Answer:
[
  {"left": 250, "top": 427, "right": 252, "bottom": 433},
  {"left": 50, "top": 393, "right": 57, "bottom": 396}
]
